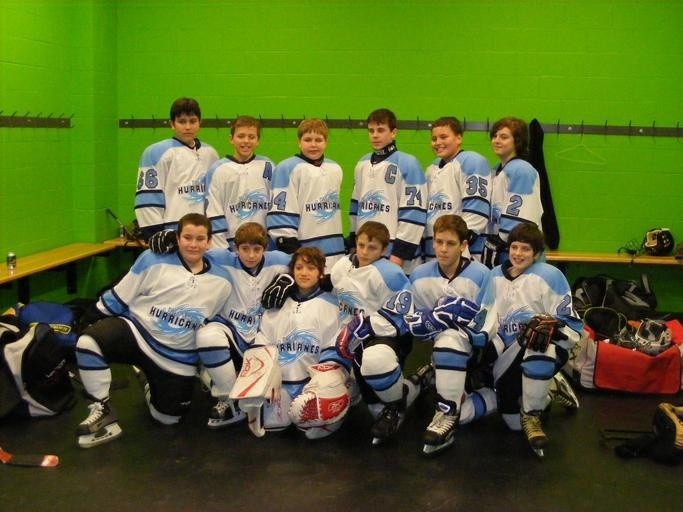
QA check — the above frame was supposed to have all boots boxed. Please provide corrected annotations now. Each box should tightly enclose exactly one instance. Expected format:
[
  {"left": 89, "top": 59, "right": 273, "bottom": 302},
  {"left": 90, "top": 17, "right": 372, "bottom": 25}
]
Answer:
[
  {"left": 519, "top": 395, "right": 546, "bottom": 449},
  {"left": 422, "top": 389, "right": 469, "bottom": 445},
  {"left": 548, "top": 372, "right": 579, "bottom": 409}
]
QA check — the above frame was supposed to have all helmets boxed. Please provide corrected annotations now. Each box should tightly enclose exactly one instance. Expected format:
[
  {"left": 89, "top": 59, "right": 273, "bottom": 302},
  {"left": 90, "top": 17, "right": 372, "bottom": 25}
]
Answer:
[
  {"left": 582, "top": 306, "right": 672, "bottom": 356},
  {"left": 644, "top": 228, "right": 673, "bottom": 256}
]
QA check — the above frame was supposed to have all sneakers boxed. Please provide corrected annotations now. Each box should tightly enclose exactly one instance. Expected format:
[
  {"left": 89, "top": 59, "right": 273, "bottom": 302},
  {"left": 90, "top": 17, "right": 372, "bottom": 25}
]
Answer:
[
  {"left": 369, "top": 404, "right": 406, "bottom": 440},
  {"left": 78, "top": 399, "right": 119, "bottom": 434},
  {"left": 209, "top": 396, "right": 238, "bottom": 419}
]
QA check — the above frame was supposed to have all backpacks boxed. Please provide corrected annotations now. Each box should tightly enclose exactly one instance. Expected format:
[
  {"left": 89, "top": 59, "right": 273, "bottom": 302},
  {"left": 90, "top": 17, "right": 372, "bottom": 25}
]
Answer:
[{"left": 0, "top": 294, "right": 104, "bottom": 419}]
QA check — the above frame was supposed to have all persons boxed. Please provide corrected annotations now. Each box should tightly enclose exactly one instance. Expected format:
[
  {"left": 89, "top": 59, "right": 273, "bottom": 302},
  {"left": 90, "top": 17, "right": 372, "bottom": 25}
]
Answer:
[
  {"left": 75, "top": 213, "right": 235, "bottom": 434},
  {"left": 136, "top": 96, "right": 585, "bottom": 450}
]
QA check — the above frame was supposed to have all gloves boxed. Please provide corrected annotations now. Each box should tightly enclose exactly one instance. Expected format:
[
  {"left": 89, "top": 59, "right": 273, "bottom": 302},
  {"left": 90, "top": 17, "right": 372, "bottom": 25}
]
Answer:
[
  {"left": 481, "top": 234, "right": 506, "bottom": 269},
  {"left": 148, "top": 229, "right": 178, "bottom": 255},
  {"left": 517, "top": 314, "right": 558, "bottom": 353},
  {"left": 335, "top": 310, "right": 374, "bottom": 361},
  {"left": 276, "top": 237, "right": 301, "bottom": 254},
  {"left": 406, "top": 308, "right": 443, "bottom": 343},
  {"left": 431, "top": 296, "right": 487, "bottom": 332},
  {"left": 260, "top": 273, "right": 295, "bottom": 309}
]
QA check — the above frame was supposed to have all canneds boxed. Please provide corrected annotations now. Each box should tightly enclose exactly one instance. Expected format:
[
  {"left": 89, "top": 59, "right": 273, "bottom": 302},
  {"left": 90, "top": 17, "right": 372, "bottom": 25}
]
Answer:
[{"left": 6, "top": 252, "right": 16, "bottom": 271}]
